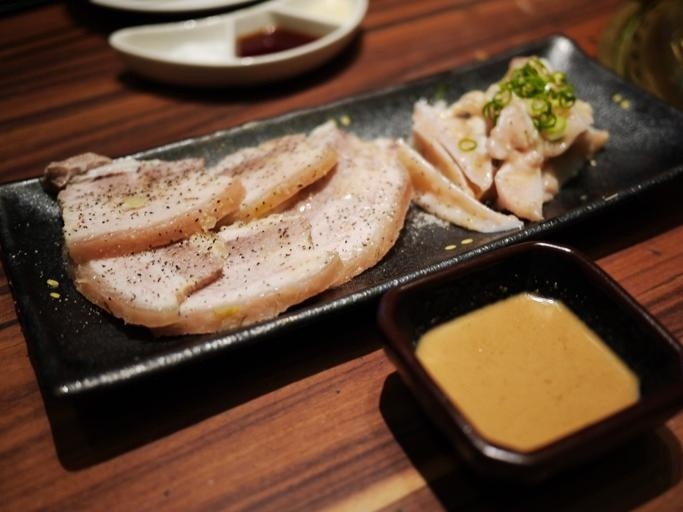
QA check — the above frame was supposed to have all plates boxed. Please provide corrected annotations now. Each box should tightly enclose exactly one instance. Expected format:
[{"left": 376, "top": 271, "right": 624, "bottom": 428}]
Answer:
[
  {"left": 107, "top": 2, "right": 368, "bottom": 79},
  {"left": 0, "top": 34, "right": 681, "bottom": 404}
]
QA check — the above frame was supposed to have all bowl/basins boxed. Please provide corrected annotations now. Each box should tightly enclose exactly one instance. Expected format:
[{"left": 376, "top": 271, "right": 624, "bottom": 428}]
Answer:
[{"left": 377, "top": 236, "right": 683, "bottom": 472}]
